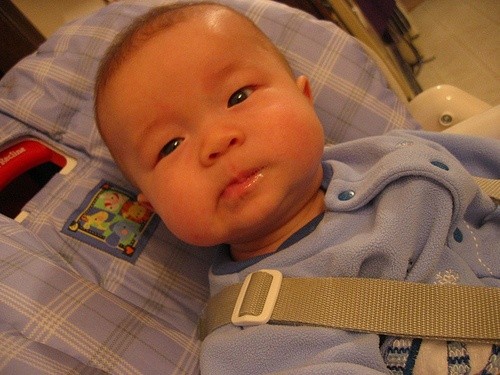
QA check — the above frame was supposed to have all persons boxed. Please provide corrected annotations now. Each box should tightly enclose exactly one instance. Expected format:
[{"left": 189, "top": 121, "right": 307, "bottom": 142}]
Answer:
[{"left": 96, "top": 1, "right": 500, "bottom": 375}]
